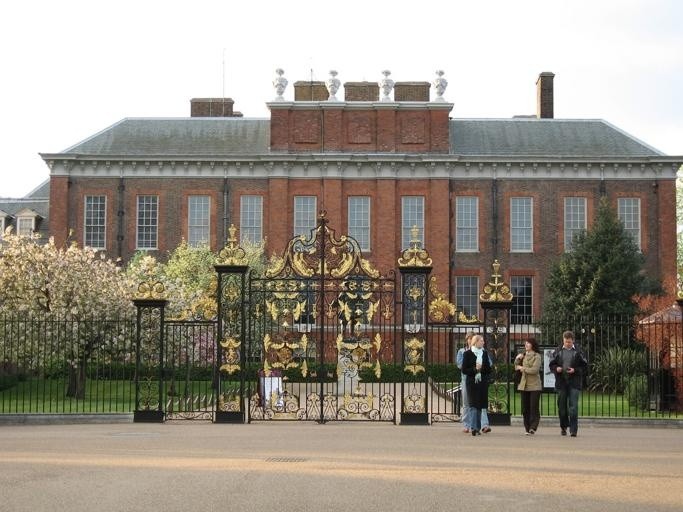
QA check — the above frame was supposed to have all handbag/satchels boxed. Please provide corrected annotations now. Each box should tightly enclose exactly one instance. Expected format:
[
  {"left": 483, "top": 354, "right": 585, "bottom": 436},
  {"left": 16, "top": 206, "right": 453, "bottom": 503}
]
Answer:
[{"left": 514, "top": 370, "right": 522, "bottom": 383}]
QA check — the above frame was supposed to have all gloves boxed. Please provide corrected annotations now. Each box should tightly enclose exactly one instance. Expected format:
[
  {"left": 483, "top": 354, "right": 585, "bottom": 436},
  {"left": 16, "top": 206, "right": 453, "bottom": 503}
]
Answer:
[{"left": 475, "top": 373, "right": 481, "bottom": 384}]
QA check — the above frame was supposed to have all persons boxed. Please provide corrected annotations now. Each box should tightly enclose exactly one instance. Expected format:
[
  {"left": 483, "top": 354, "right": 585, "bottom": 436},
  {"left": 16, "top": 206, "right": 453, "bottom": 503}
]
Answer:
[
  {"left": 512, "top": 337, "right": 543, "bottom": 436},
  {"left": 460, "top": 334, "right": 492, "bottom": 436},
  {"left": 547, "top": 331, "right": 588, "bottom": 437},
  {"left": 454, "top": 329, "right": 492, "bottom": 434}
]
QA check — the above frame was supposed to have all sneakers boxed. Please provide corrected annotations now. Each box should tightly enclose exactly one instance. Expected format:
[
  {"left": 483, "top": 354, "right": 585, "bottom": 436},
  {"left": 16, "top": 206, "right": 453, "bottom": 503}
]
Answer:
[
  {"left": 463, "top": 427, "right": 491, "bottom": 436},
  {"left": 561, "top": 428, "right": 577, "bottom": 436},
  {"left": 525, "top": 429, "right": 535, "bottom": 435}
]
